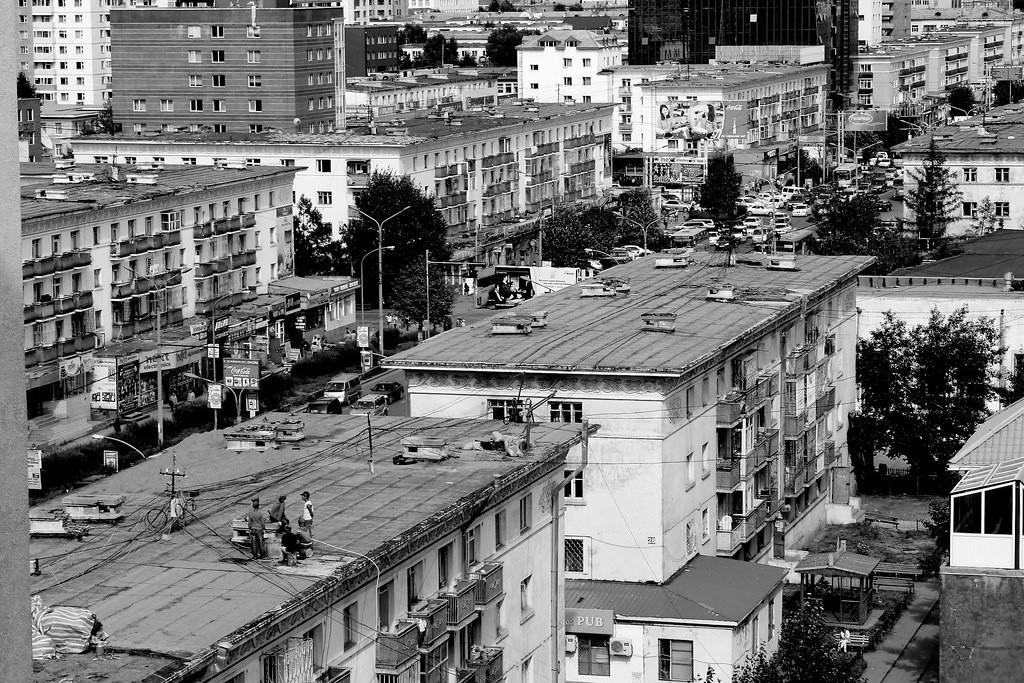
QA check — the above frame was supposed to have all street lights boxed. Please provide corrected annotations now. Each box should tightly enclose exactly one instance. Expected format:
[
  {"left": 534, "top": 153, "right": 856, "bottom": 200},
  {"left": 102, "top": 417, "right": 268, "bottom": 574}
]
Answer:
[
  {"left": 361, "top": 245, "right": 395, "bottom": 327},
  {"left": 611, "top": 209, "right": 679, "bottom": 256},
  {"left": 348, "top": 204, "right": 411, "bottom": 364},
  {"left": 183, "top": 366, "right": 289, "bottom": 427},
  {"left": 513, "top": 167, "right": 568, "bottom": 267},
  {"left": 122, "top": 265, "right": 194, "bottom": 447},
  {"left": 212, "top": 289, "right": 252, "bottom": 382},
  {"left": 781, "top": 103, "right": 815, "bottom": 186}
]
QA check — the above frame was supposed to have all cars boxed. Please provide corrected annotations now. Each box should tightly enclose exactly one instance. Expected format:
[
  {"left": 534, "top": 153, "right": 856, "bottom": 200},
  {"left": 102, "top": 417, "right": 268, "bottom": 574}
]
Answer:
[{"left": 474, "top": 150, "right": 912, "bottom": 311}]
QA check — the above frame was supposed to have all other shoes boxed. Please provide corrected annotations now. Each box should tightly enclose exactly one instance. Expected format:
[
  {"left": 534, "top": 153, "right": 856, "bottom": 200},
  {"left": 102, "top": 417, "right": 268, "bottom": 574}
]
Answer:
[
  {"left": 278, "top": 559, "right": 287, "bottom": 563},
  {"left": 263, "top": 556, "right": 270, "bottom": 559},
  {"left": 252, "top": 555, "right": 259, "bottom": 560}
]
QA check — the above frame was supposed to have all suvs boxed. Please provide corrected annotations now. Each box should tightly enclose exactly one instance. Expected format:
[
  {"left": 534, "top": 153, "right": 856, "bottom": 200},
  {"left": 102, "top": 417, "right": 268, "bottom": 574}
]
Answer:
[{"left": 306, "top": 372, "right": 405, "bottom": 416}]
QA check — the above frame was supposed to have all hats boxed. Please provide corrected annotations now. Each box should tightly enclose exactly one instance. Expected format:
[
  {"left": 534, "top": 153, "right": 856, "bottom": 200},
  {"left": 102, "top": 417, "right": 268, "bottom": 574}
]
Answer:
[
  {"left": 300, "top": 491, "right": 310, "bottom": 496},
  {"left": 285, "top": 525, "right": 291, "bottom": 530}
]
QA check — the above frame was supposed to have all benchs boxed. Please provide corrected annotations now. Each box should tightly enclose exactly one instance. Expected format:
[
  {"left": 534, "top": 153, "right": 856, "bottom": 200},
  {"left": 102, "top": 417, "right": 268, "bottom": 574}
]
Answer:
[
  {"left": 865, "top": 512, "right": 900, "bottom": 529},
  {"left": 873, "top": 576, "right": 915, "bottom": 594},
  {"left": 872, "top": 561, "right": 923, "bottom": 581},
  {"left": 832, "top": 634, "right": 869, "bottom": 658}
]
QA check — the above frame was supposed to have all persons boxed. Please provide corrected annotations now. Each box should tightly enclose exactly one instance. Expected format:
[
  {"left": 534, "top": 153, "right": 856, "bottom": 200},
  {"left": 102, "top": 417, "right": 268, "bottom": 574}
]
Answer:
[
  {"left": 187, "top": 389, "right": 195, "bottom": 403},
  {"left": 169, "top": 392, "right": 178, "bottom": 411},
  {"left": 298, "top": 278, "right": 479, "bottom": 354},
  {"left": 300, "top": 491, "right": 313, "bottom": 535},
  {"left": 277, "top": 525, "right": 296, "bottom": 562},
  {"left": 494, "top": 275, "right": 532, "bottom": 303},
  {"left": 95, "top": 402, "right": 126, "bottom": 435},
  {"left": 248, "top": 502, "right": 266, "bottom": 559},
  {"left": 269, "top": 495, "right": 289, "bottom": 532},
  {"left": 296, "top": 519, "right": 313, "bottom": 559}
]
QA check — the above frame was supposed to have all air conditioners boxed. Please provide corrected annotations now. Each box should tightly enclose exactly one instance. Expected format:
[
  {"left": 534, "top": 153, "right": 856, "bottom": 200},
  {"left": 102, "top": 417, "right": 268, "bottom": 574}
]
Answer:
[
  {"left": 565, "top": 635, "right": 577, "bottom": 654},
  {"left": 609, "top": 637, "right": 634, "bottom": 658}
]
